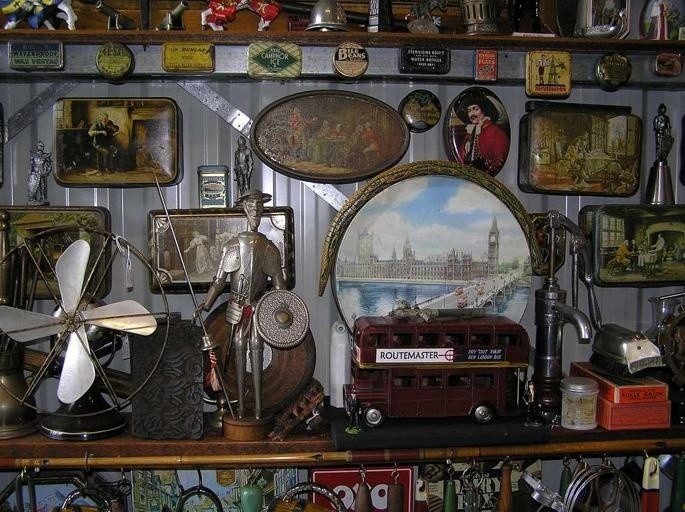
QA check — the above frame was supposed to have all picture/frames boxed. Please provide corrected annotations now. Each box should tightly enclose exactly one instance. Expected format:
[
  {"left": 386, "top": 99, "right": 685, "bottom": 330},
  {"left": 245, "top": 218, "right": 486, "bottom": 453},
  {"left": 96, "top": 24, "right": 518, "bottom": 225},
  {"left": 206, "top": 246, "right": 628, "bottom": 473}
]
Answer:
[
  {"left": 317, "top": 158, "right": 544, "bottom": 340},
  {"left": 147, "top": 205, "right": 297, "bottom": 294},
  {"left": 0, "top": 206, "right": 112, "bottom": 300},
  {"left": 249, "top": 89, "right": 411, "bottom": 186}
]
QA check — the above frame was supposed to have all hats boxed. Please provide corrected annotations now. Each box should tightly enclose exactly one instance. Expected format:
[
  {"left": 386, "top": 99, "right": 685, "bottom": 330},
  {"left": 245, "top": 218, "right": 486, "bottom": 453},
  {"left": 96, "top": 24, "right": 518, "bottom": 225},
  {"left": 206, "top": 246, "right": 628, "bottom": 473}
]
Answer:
[{"left": 454, "top": 92, "right": 499, "bottom": 124}]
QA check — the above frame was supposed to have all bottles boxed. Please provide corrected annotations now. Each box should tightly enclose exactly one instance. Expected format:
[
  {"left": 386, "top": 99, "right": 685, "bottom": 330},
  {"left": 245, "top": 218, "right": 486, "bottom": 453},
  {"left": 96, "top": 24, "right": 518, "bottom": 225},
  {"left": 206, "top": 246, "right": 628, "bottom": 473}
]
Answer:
[{"left": 644, "top": 157, "right": 675, "bottom": 205}]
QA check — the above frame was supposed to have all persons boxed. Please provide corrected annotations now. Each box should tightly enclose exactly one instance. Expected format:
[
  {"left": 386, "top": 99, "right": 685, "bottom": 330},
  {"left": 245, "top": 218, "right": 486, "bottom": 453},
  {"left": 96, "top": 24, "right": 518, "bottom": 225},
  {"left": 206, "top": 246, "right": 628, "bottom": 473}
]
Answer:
[
  {"left": 653, "top": 103, "right": 672, "bottom": 161},
  {"left": 27, "top": 140, "right": 52, "bottom": 207},
  {"left": 281, "top": 105, "right": 381, "bottom": 171},
  {"left": 89, "top": 112, "right": 119, "bottom": 173},
  {"left": 461, "top": 104, "right": 507, "bottom": 174},
  {"left": 536, "top": 54, "right": 550, "bottom": 85},
  {"left": 234, "top": 135, "right": 255, "bottom": 197},
  {"left": 191, "top": 187, "right": 285, "bottom": 420},
  {"left": 183, "top": 231, "right": 213, "bottom": 275}
]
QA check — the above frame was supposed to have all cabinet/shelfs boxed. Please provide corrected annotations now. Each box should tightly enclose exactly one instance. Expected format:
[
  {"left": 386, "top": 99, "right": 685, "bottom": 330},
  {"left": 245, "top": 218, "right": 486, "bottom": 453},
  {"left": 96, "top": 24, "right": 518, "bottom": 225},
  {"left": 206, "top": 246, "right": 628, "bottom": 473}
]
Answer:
[{"left": 1, "top": 25, "right": 685, "bottom": 512}]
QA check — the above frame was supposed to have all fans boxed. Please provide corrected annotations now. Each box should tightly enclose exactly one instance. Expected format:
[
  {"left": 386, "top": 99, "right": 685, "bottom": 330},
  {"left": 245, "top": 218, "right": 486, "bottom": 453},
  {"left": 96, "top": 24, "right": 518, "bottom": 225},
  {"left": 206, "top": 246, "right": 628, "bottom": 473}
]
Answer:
[{"left": 0, "top": 228, "right": 170, "bottom": 442}]
